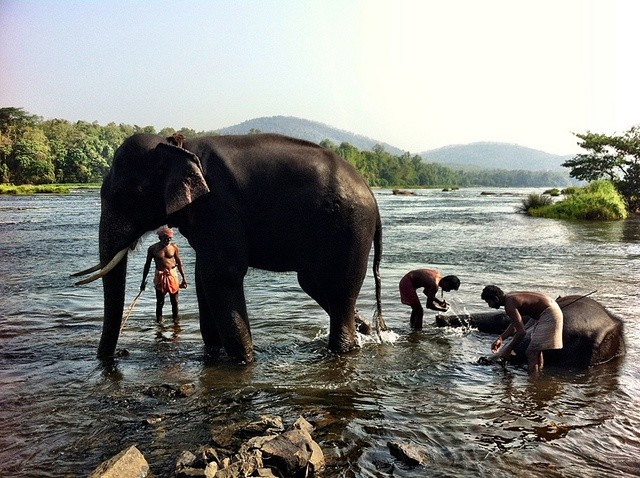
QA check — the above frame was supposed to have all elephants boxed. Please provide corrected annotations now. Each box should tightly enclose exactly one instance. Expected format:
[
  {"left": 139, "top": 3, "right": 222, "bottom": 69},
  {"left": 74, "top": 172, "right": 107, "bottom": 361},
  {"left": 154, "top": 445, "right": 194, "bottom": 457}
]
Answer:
[
  {"left": 69, "top": 132, "right": 388, "bottom": 367},
  {"left": 436, "top": 295, "right": 626, "bottom": 370}
]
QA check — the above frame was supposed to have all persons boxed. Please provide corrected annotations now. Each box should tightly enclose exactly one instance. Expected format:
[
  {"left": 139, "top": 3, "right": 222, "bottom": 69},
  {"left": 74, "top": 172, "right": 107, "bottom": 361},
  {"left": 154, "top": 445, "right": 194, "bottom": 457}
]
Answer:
[
  {"left": 139, "top": 228, "right": 187, "bottom": 323},
  {"left": 398, "top": 268, "right": 460, "bottom": 330},
  {"left": 480, "top": 284, "right": 564, "bottom": 376}
]
